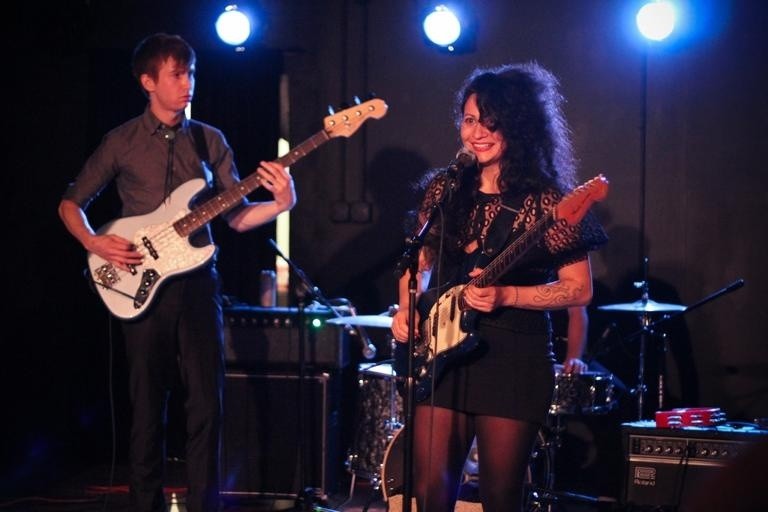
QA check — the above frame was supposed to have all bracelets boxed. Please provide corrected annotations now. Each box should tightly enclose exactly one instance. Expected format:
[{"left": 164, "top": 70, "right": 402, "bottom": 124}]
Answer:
[{"left": 512, "top": 286, "right": 519, "bottom": 307}]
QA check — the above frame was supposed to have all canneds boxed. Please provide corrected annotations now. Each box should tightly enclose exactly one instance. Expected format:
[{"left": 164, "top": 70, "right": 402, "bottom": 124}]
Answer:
[{"left": 259, "top": 270, "right": 276, "bottom": 307}]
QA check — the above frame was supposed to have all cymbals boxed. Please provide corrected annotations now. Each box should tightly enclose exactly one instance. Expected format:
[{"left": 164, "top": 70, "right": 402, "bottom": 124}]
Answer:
[
  {"left": 596, "top": 295, "right": 688, "bottom": 315},
  {"left": 324, "top": 315, "right": 394, "bottom": 329}
]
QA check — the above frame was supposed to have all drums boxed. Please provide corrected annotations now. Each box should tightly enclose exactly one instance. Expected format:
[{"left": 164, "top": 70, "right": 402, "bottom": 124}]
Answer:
[
  {"left": 346, "top": 362, "right": 412, "bottom": 490},
  {"left": 547, "top": 370, "right": 613, "bottom": 415},
  {"left": 377, "top": 422, "right": 556, "bottom": 512}
]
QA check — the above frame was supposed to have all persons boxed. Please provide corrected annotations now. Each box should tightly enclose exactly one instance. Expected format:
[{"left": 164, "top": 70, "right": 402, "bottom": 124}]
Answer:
[
  {"left": 56, "top": 32, "right": 297, "bottom": 512},
  {"left": 563, "top": 305, "right": 589, "bottom": 376},
  {"left": 389, "top": 58, "right": 597, "bottom": 512}
]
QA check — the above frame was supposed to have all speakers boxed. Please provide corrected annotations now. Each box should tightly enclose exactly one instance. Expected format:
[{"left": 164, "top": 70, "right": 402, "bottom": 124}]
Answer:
[
  {"left": 217, "top": 372, "right": 353, "bottom": 499},
  {"left": 620, "top": 421, "right": 768, "bottom": 511}
]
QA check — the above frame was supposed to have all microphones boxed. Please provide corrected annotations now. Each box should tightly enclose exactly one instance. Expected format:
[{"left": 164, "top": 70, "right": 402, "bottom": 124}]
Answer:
[
  {"left": 585, "top": 322, "right": 617, "bottom": 364},
  {"left": 447, "top": 145, "right": 478, "bottom": 183},
  {"left": 349, "top": 303, "right": 377, "bottom": 360}
]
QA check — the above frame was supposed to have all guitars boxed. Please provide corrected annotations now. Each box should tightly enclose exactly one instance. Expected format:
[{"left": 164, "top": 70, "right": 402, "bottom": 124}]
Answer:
[
  {"left": 391, "top": 172, "right": 611, "bottom": 402},
  {"left": 86, "top": 86, "right": 388, "bottom": 321}
]
